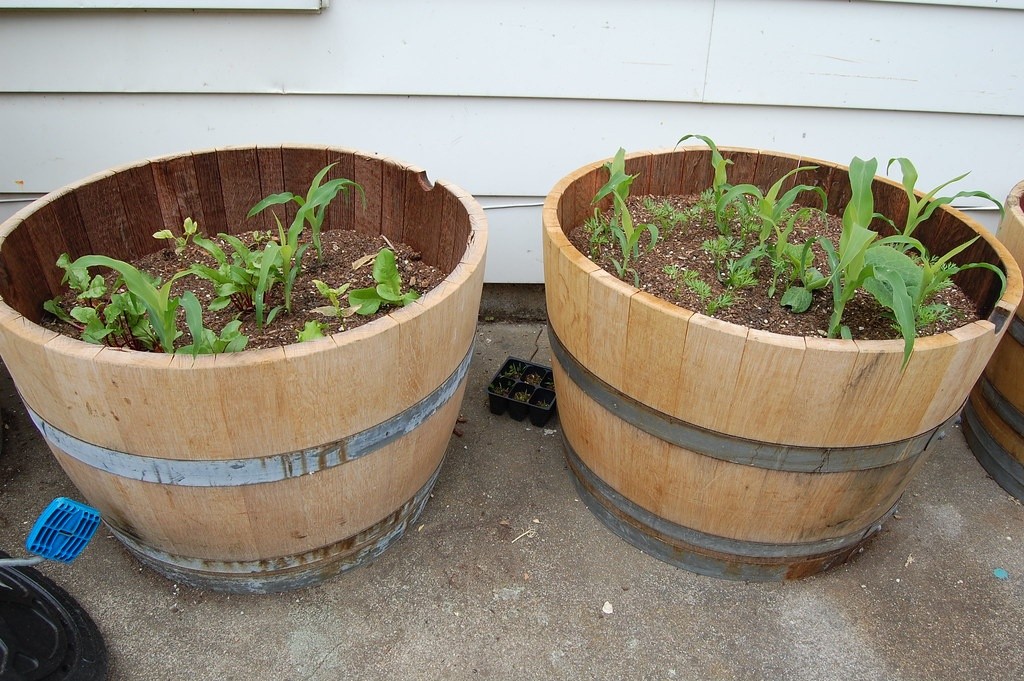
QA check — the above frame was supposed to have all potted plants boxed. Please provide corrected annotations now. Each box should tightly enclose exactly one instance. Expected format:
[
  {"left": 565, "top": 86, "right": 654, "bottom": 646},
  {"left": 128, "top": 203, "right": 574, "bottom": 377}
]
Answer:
[
  {"left": 0, "top": 143, "right": 489, "bottom": 597},
  {"left": 540, "top": 130, "right": 1024, "bottom": 582},
  {"left": 485, "top": 355, "right": 559, "bottom": 428}
]
